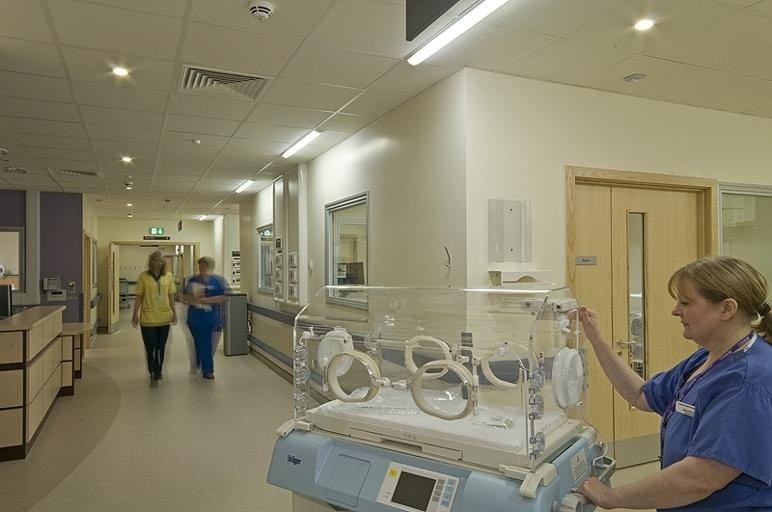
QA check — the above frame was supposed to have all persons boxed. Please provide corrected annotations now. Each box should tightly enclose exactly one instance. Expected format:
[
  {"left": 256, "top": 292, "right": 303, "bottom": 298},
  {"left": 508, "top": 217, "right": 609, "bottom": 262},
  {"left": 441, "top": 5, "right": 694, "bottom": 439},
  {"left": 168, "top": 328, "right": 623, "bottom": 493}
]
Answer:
[
  {"left": 178, "top": 256, "right": 232, "bottom": 381},
  {"left": 131, "top": 251, "right": 180, "bottom": 390},
  {"left": 578, "top": 253, "right": 772, "bottom": 512}
]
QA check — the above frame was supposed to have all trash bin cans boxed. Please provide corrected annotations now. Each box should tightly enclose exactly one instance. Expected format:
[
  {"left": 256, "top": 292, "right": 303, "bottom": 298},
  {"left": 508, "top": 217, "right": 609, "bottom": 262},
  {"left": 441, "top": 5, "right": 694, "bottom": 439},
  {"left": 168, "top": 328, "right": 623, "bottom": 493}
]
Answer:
[{"left": 223, "top": 293, "right": 248, "bottom": 356}]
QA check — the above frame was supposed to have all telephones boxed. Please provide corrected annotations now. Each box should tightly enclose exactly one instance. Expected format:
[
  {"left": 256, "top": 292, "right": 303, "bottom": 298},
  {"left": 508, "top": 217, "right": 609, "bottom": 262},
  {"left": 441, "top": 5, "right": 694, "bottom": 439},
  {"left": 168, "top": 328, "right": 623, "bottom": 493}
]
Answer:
[{"left": 43, "top": 278, "right": 62, "bottom": 291}]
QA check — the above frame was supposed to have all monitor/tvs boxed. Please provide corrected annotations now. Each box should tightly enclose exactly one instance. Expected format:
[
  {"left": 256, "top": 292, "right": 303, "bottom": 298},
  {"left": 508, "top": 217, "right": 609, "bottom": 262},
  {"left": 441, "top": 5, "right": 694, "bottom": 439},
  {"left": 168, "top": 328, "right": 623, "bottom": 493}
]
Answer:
[{"left": 0, "top": 284, "right": 12, "bottom": 319}]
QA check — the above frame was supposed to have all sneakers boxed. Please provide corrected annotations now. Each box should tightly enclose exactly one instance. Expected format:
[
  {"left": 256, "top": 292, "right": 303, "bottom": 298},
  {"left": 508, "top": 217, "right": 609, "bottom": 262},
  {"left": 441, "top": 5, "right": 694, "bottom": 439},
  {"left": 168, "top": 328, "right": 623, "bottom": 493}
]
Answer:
[
  {"left": 203, "top": 373, "right": 215, "bottom": 377},
  {"left": 149, "top": 381, "right": 157, "bottom": 387}
]
